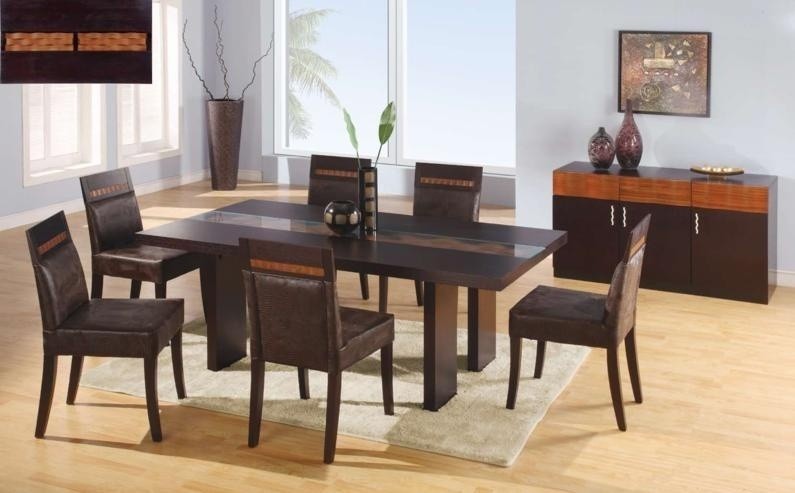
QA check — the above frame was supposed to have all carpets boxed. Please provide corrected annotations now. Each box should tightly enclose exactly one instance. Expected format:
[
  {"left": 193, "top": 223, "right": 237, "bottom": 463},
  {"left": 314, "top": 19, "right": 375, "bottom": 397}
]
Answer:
[{"left": 77, "top": 309, "right": 588, "bottom": 468}]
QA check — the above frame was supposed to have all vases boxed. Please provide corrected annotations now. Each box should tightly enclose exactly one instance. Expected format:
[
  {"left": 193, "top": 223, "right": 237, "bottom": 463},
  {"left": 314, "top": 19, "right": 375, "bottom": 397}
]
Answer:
[
  {"left": 615, "top": 98, "right": 643, "bottom": 169},
  {"left": 207, "top": 97, "right": 243, "bottom": 191},
  {"left": 587, "top": 126, "right": 615, "bottom": 169}
]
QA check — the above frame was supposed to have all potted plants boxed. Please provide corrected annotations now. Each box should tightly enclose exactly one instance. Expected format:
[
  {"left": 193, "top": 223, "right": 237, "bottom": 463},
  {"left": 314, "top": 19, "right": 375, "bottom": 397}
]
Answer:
[{"left": 342, "top": 102, "right": 398, "bottom": 230}]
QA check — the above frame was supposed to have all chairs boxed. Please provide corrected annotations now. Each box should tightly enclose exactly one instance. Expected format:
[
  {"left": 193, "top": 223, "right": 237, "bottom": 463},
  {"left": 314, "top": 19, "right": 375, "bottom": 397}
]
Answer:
[
  {"left": 24, "top": 211, "right": 185, "bottom": 441},
  {"left": 79, "top": 167, "right": 199, "bottom": 345},
  {"left": 380, "top": 163, "right": 482, "bottom": 307},
  {"left": 238, "top": 236, "right": 394, "bottom": 464},
  {"left": 310, "top": 154, "right": 374, "bottom": 300},
  {"left": 505, "top": 212, "right": 649, "bottom": 432}
]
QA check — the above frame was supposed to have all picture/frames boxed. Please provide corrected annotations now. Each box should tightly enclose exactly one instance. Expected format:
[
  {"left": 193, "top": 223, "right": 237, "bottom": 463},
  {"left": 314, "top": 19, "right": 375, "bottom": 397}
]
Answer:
[{"left": 618, "top": 31, "right": 712, "bottom": 117}]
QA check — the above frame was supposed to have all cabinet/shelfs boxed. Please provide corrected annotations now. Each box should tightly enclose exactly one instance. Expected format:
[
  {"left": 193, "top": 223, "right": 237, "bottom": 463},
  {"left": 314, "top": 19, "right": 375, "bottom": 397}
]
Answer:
[
  {"left": 692, "top": 175, "right": 778, "bottom": 305},
  {"left": 552, "top": 162, "right": 692, "bottom": 294}
]
